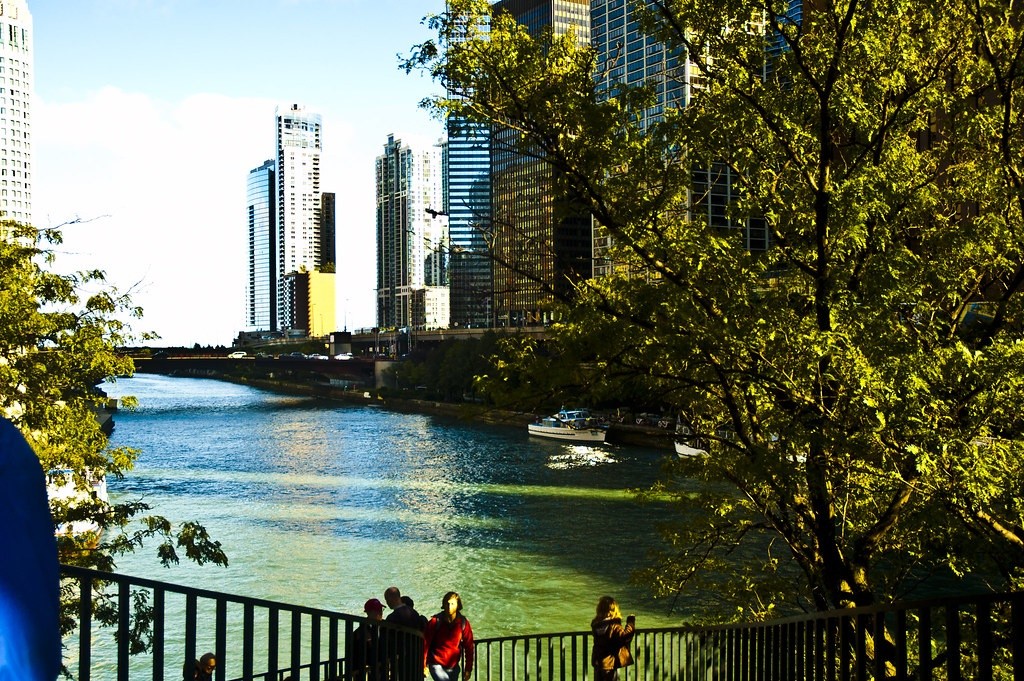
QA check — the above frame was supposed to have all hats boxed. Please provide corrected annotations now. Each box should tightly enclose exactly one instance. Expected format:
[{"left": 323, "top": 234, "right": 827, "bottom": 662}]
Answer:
[{"left": 364, "top": 599, "right": 387, "bottom": 613}]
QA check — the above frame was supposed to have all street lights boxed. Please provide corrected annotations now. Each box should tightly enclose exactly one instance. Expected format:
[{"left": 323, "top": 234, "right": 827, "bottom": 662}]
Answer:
[
  {"left": 487, "top": 297, "right": 491, "bottom": 327},
  {"left": 312, "top": 304, "right": 316, "bottom": 335}
]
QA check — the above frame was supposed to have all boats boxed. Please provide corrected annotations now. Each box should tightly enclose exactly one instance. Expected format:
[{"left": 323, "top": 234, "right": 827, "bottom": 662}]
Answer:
[
  {"left": 528, "top": 409, "right": 610, "bottom": 441},
  {"left": 673, "top": 424, "right": 710, "bottom": 459},
  {"left": 45, "top": 466, "right": 114, "bottom": 544},
  {"left": 342, "top": 389, "right": 383, "bottom": 403}
]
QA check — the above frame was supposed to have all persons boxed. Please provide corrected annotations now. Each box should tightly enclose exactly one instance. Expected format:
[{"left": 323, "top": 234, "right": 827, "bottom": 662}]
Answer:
[
  {"left": 590, "top": 596, "right": 635, "bottom": 681},
  {"left": 353, "top": 587, "right": 474, "bottom": 681},
  {"left": 182, "top": 652, "right": 216, "bottom": 681}
]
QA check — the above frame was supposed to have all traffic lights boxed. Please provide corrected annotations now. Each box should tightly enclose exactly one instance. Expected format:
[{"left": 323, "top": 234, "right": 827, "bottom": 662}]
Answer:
[{"left": 385, "top": 346, "right": 389, "bottom": 356}]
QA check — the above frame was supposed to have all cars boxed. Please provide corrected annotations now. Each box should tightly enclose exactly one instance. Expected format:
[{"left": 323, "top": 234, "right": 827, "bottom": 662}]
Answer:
[
  {"left": 151, "top": 351, "right": 169, "bottom": 358},
  {"left": 290, "top": 352, "right": 308, "bottom": 359},
  {"left": 304, "top": 354, "right": 329, "bottom": 360},
  {"left": 513, "top": 410, "right": 523, "bottom": 414},
  {"left": 544, "top": 320, "right": 560, "bottom": 327},
  {"left": 334, "top": 354, "right": 354, "bottom": 360},
  {"left": 560, "top": 402, "right": 593, "bottom": 412},
  {"left": 635, "top": 414, "right": 660, "bottom": 425}
]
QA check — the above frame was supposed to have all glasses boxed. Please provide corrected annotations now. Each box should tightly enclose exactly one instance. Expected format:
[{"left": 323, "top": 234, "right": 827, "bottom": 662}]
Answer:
[{"left": 206, "top": 664, "right": 215, "bottom": 670}]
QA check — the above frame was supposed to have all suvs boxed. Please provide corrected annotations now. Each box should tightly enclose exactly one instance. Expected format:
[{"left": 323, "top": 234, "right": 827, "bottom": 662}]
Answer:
[{"left": 228, "top": 352, "right": 247, "bottom": 359}]
